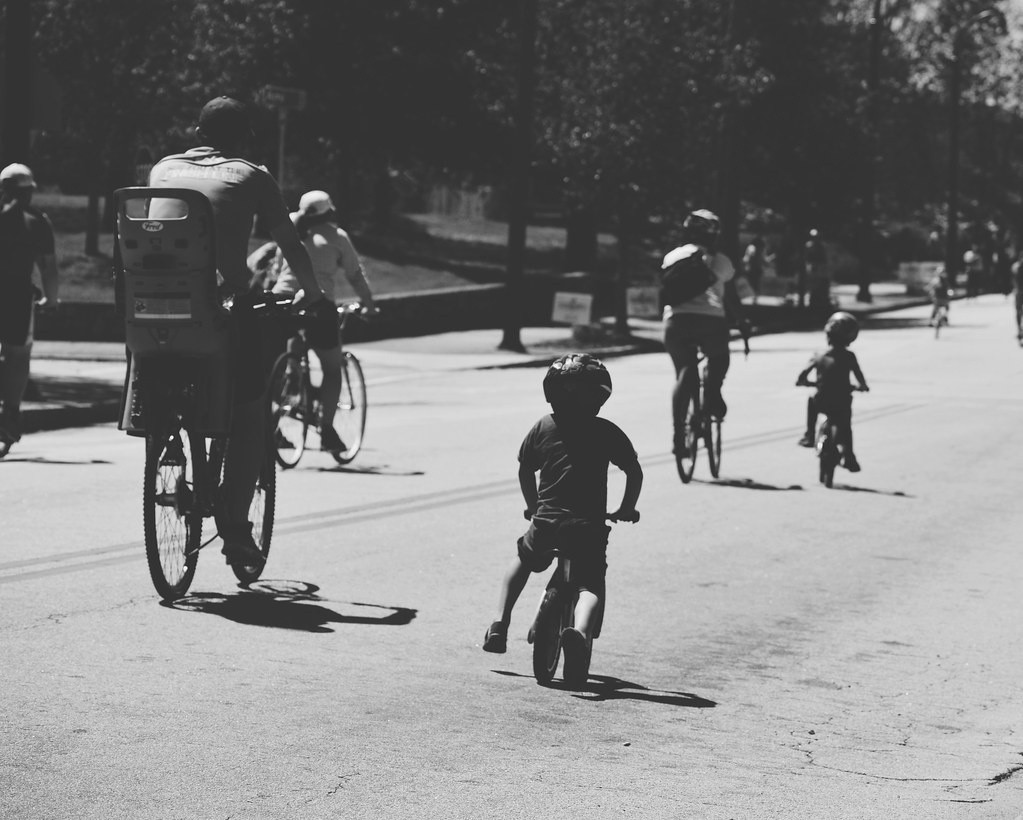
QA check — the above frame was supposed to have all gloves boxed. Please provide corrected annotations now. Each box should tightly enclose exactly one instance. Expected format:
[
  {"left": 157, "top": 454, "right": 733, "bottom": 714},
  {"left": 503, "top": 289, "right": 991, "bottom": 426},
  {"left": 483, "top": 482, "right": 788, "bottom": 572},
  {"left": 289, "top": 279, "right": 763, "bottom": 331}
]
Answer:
[
  {"left": 233, "top": 282, "right": 276, "bottom": 319},
  {"left": 304, "top": 290, "right": 339, "bottom": 325}
]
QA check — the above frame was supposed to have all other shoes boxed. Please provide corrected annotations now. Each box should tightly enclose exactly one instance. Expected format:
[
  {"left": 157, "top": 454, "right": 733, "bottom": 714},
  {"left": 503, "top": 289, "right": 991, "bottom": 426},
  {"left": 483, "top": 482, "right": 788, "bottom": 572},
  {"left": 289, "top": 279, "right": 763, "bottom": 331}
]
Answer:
[
  {"left": 220, "top": 523, "right": 267, "bottom": 565},
  {"left": 271, "top": 428, "right": 294, "bottom": 449},
  {"left": 845, "top": 452, "right": 862, "bottom": 473},
  {"left": 321, "top": 430, "right": 346, "bottom": 452},
  {"left": 484, "top": 621, "right": 508, "bottom": 654},
  {"left": 0, "top": 408, "right": 22, "bottom": 442},
  {"left": 799, "top": 436, "right": 818, "bottom": 449},
  {"left": 707, "top": 391, "right": 727, "bottom": 420},
  {"left": 560, "top": 625, "right": 589, "bottom": 691}
]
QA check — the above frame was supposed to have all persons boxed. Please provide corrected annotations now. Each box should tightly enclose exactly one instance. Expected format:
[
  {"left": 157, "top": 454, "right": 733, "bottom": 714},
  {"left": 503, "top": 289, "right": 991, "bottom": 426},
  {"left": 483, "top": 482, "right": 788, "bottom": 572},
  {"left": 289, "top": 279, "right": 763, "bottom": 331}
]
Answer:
[
  {"left": 774, "top": 226, "right": 799, "bottom": 306},
  {"left": 798, "top": 311, "right": 868, "bottom": 471},
  {"left": 928, "top": 265, "right": 949, "bottom": 326},
  {"left": 964, "top": 244, "right": 984, "bottom": 299},
  {"left": 924, "top": 226, "right": 946, "bottom": 258},
  {"left": 1, "top": 162, "right": 59, "bottom": 458},
  {"left": 484, "top": 354, "right": 642, "bottom": 690},
  {"left": 1004, "top": 251, "right": 1023, "bottom": 346},
  {"left": 743, "top": 238, "right": 766, "bottom": 307},
  {"left": 138, "top": 96, "right": 381, "bottom": 567},
  {"left": 658, "top": 208, "right": 750, "bottom": 457}
]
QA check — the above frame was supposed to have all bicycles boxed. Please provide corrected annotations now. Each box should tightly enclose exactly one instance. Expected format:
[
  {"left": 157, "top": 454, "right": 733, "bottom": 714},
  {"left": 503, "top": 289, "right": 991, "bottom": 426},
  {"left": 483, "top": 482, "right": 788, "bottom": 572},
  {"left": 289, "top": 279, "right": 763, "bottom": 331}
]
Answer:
[
  {"left": 671, "top": 321, "right": 754, "bottom": 483},
  {"left": 0, "top": 286, "right": 65, "bottom": 461},
  {"left": 106, "top": 183, "right": 331, "bottom": 602},
  {"left": 796, "top": 379, "right": 868, "bottom": 491},
  {"left": 263, "top": 306, "right": 375, "bottom": 468},
  {"left": 520, "top": 511, "right": 641, "bottom": 686}
]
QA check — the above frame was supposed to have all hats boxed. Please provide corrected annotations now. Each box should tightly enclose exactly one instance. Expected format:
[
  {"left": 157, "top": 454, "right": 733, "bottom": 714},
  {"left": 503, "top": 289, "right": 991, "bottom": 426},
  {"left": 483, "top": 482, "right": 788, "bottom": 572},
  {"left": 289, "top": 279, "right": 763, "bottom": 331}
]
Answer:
[
  {"left": 299, "top": 189, "right": 336, "bottom": 217},
  {"left": 199, "top": 98, "right": 254, "bottom": 143},
  {"left": 0, "top": 163, "right": 37, "bottom": 190}
]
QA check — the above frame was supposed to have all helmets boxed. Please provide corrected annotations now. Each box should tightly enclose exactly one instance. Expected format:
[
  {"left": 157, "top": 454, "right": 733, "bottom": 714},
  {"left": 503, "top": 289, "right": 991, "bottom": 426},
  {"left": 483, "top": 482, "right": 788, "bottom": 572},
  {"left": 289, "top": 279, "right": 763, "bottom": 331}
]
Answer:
[
  {"left": 682, "top": 209, "right": 721, "bottom": 239},
  {"left": 825, "top": 312, "right": 858, "bottom": 347},
  {"left": 541, "top": 351, "right": 613, "bottom": 410}
]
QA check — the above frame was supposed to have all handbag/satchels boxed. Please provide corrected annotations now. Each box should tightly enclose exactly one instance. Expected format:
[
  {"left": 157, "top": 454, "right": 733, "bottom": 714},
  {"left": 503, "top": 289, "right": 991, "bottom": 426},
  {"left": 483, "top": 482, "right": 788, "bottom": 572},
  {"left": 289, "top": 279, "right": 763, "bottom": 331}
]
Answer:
[{"left": 660, "top": 250, "right": 715, "bottom": 306}]
